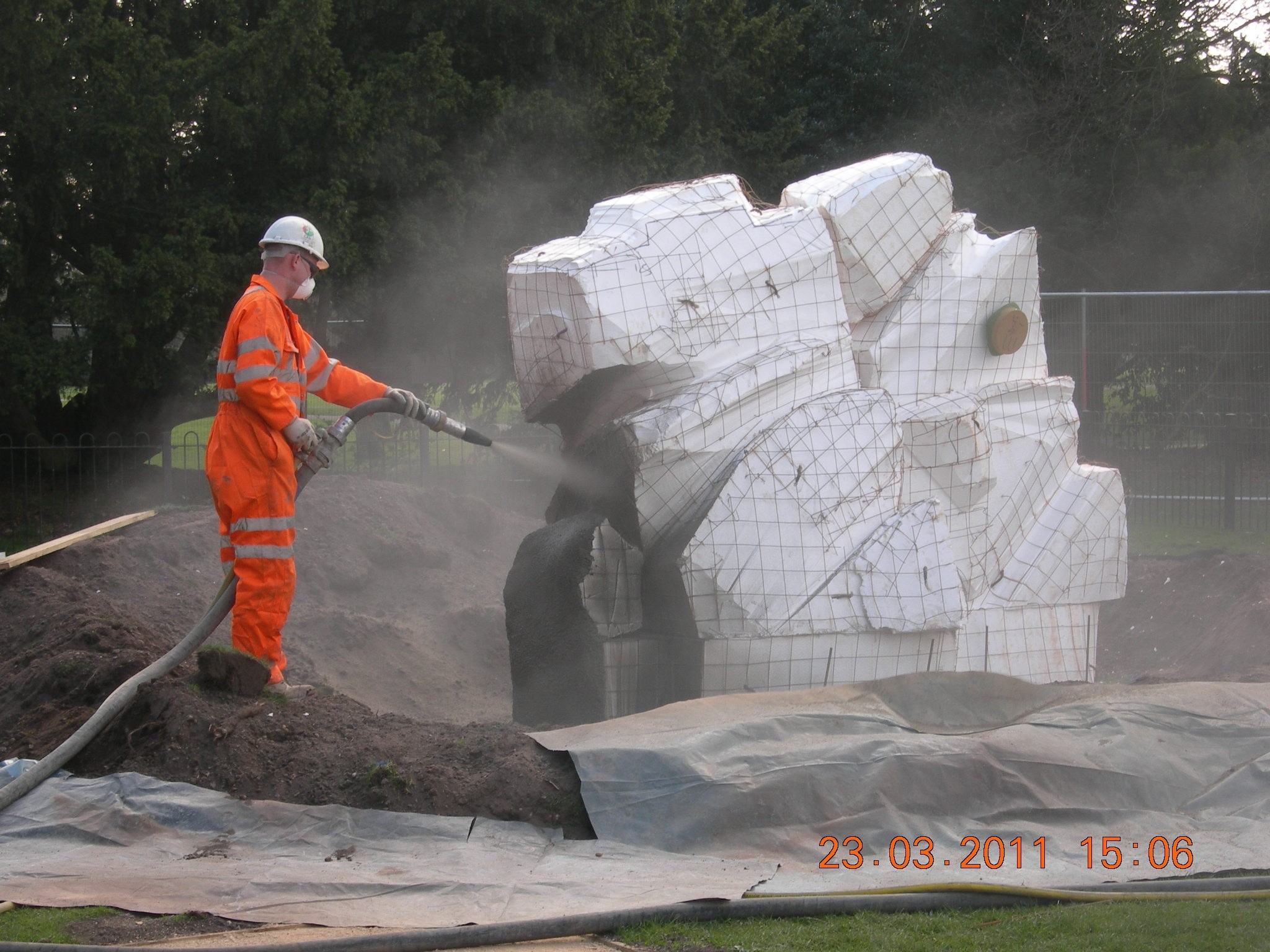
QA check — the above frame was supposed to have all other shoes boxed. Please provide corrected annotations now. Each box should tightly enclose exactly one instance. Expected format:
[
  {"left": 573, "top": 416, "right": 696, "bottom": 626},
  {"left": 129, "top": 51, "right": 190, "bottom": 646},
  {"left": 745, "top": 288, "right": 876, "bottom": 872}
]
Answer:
[{"left": 265, "top": 680, "right": 316, "bottom": 702}]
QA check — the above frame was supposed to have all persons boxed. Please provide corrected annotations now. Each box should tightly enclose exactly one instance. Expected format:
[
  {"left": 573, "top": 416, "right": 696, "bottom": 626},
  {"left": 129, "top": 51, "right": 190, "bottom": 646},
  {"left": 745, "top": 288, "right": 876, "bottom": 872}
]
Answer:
[{"left": 203, "top": 212, "right": 431, "bottom": 688}]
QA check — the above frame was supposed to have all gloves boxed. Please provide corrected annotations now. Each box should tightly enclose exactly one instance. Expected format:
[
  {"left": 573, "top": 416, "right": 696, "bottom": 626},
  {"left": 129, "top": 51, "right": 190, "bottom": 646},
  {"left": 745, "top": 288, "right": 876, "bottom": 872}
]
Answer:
[
  {"left": 283, "top": 415, "right": 318, "bottom": 453},
  {"left": 385, "top": 385, "right": 429, "bottom": 419}
]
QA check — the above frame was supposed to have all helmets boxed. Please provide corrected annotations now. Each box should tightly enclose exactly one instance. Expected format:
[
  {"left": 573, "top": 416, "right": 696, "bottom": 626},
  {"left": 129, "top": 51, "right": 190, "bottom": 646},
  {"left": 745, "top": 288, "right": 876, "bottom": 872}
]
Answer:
[{"left": 259, "top": 216, "right": 330, "bottom": 270}]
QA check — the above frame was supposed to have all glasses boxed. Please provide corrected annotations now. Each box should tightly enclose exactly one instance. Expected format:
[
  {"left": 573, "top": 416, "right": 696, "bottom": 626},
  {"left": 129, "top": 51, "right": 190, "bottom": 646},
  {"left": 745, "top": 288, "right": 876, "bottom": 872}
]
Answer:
[{"left": 290, "top": 251, "right": 319, "bottom": 278}]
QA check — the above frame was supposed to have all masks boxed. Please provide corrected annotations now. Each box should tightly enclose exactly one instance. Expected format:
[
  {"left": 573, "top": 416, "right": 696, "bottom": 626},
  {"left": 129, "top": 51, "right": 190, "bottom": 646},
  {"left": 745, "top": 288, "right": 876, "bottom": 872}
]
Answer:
[{"left": 294, "top": 279, "right": 316, "bottom": 300}]
